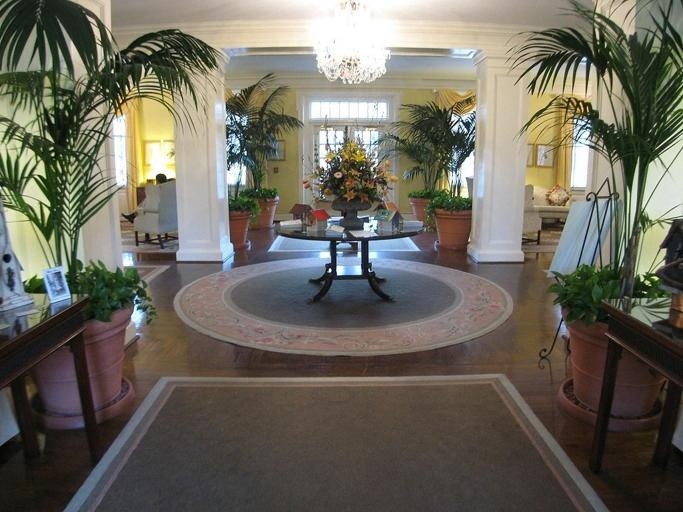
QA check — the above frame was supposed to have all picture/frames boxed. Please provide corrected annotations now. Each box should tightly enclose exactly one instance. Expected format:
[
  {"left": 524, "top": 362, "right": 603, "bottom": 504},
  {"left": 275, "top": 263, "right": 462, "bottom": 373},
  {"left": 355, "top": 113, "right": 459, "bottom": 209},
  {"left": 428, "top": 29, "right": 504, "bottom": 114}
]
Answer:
[
  {"left": 535, "top": 144, "right": 554, "bottom": 168},
  {"left": 266, "top": 140, "right": 286, "bottom": 162},
  {"left": 525, "top": 143, "right": 534, "bottom": 168}
]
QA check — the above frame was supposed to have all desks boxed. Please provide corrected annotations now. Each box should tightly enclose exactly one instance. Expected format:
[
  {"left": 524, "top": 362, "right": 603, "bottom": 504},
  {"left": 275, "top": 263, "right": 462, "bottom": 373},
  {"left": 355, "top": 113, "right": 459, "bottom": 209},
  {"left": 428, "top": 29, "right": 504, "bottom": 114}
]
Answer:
[
  {"left": 587, "top": 291, "right": 682, "bottom": 512},
  {"left": 0, "top": 292, "right": 103, "bottom": 512},
  {"left": 274, "top": 216, "right": 424, "bottom": 305}
]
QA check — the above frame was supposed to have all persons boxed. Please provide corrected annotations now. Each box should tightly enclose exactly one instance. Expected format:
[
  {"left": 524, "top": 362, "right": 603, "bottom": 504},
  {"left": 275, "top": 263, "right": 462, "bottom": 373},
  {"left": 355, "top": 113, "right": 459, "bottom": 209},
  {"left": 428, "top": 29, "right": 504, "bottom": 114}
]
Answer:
[{"left": 120, "top": 174, "right": 166, "bottom": 223}]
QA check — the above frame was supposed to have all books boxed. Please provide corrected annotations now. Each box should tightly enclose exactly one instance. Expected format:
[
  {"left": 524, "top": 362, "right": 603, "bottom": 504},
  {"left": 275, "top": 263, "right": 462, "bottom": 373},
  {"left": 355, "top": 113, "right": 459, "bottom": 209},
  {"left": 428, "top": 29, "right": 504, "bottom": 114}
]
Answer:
[
  {"left": 325, "top": 224, "right": 345, "bottom": 237},
  {"left": 278, "top": 218, "right": 302, "bottom": 232}
]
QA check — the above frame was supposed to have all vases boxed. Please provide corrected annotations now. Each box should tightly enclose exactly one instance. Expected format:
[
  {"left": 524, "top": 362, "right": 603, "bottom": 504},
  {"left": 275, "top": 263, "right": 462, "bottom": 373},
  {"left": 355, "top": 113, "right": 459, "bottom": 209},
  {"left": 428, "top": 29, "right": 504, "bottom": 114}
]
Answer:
[
  {"left": 501, "top": 2, "right": 682, "bottom": 430},
  {"left": 330, "top": 197, "right": 371, "bottom": 229}
]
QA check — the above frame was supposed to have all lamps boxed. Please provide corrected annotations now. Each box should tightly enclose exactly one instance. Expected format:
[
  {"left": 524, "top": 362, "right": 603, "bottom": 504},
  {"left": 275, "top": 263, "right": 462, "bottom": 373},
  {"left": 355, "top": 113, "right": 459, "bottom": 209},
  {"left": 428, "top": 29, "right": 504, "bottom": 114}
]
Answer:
[{"left": 307, "top": 0, "right": 389, "bottom": 84}]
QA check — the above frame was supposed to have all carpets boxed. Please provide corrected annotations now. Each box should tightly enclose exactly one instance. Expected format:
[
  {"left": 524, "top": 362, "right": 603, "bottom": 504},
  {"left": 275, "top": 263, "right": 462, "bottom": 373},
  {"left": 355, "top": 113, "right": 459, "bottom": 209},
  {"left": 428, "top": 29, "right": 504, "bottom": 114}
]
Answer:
[
  {"left": 267, "top": 219, "right": 422, "bottom": 253},
  {"left": 57, "top": 373, "right": 611, "bottom": 510},
  {"left": 171, "top": 255, "right": 515, "bottom": 358},
  {"left": 121, "top": 264, "right": 170, "bottom": 290}
]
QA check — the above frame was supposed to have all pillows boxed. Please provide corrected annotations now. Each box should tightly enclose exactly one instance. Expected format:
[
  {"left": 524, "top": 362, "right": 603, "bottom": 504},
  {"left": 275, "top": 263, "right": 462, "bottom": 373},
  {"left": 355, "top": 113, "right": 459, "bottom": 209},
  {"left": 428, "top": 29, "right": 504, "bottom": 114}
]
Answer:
[{"left": 546, "top": 184, "right": 570, "bottom": 207}]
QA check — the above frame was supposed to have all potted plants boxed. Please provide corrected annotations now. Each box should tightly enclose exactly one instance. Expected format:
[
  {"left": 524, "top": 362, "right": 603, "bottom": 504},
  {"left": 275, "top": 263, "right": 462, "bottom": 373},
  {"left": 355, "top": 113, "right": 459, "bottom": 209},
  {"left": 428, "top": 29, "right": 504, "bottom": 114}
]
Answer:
[
  {"left": 0, "top": 0, "right": 228, "bottom": 428},
  {"left": 404, "top": 98, "right": 478, "bottom": 251},
  {"left": 221, "top": 70, "right": 301, "bottom": 249},
  {"left": 370, "top": 121, "right": 449, "bottom": 227},
  {"left": 240, "top": 110, "right": 304, "bottom": 232}
]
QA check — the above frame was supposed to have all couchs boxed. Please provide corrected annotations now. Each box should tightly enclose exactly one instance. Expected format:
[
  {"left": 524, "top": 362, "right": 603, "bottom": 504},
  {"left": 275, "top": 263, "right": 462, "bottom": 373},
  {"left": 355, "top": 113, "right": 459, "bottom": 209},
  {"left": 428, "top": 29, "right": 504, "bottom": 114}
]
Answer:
[
  {"left": 527, "top": 186, "right": 572, "bottom": 226},
  {"left": 520, "top": 184, "right": 542, "bottom": 245},
  {"left": 130, "top": 177, "right": 176, "bottom": 249}
]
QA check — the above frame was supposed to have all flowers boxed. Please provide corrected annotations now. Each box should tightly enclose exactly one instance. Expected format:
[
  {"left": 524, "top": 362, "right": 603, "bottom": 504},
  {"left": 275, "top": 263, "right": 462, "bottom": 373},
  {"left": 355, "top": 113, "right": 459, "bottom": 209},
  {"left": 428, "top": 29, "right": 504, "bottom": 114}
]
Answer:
[{"left": 302, "top": 133, "right": 398, "bottom": 203}]
